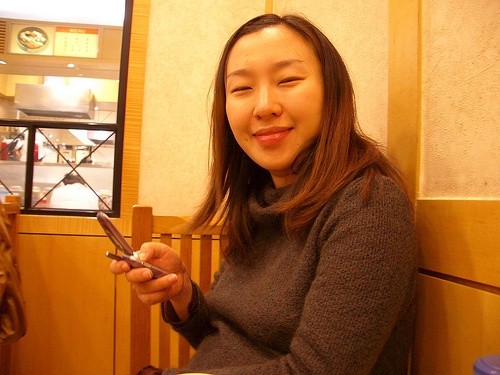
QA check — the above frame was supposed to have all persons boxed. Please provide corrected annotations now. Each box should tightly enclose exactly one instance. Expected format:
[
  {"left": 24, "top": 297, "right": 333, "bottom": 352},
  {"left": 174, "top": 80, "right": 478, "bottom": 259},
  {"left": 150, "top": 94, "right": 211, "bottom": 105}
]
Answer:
[
  {"left": 49, "top": 171, "right": 99, "bottom": 210},
  {"left": 110, "top": 13, "right": 417, "bottom": 375}
]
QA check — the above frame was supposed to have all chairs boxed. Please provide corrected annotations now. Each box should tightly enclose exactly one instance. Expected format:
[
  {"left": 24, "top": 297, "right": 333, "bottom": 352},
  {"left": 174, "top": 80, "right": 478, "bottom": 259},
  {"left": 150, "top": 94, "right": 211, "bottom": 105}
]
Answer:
[{"left": 130, "top": 205, "right": 229, "bottom": 375}]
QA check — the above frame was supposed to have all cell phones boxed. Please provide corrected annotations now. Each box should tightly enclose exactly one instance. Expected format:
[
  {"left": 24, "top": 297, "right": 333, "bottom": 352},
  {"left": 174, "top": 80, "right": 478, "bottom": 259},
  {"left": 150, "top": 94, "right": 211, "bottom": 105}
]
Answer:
[{"left": 97, "top": 212, "right": 168, "bottom": 279}]
